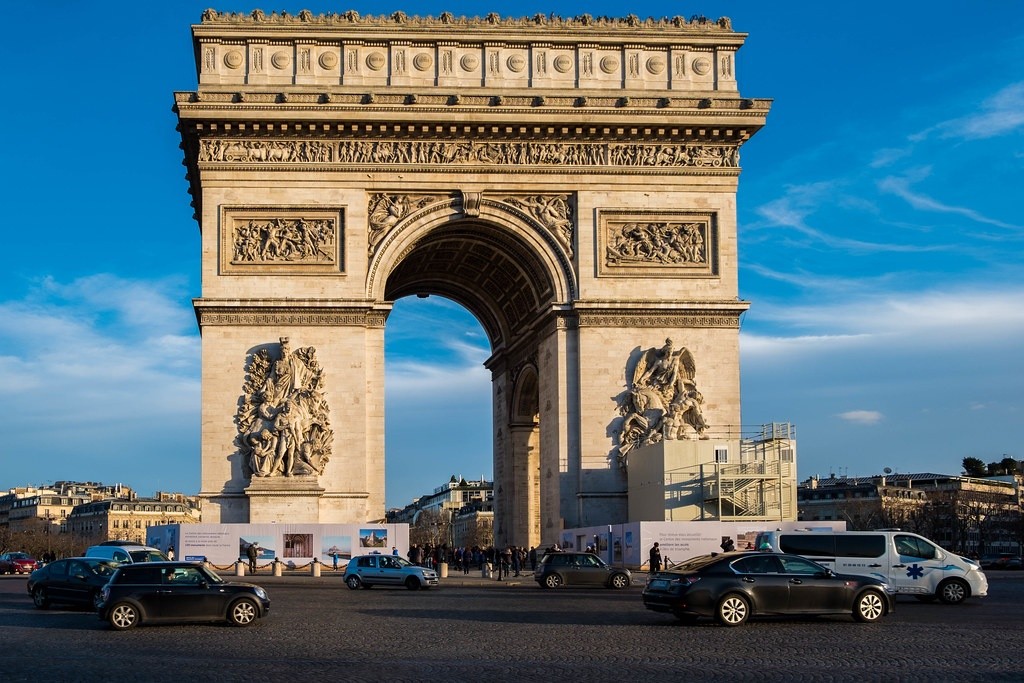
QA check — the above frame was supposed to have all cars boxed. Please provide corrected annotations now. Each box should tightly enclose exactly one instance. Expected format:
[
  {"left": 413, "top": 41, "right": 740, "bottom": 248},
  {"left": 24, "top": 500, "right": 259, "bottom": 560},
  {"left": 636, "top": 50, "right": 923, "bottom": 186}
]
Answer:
[
  {"left": 0, "top": 552, "right": 39, "bottom": 574},
  {"left": 343, "top": 554, "right": 439, "bottom": 590},
  {"left": 27, "top": 540, "right": 271, "bottom": 631},
  {"left": 534, "top": 552, "right": 633, "bottom": 589},
  {"left": 642, "top": 527, "right": 1024, "bottom": 627}
]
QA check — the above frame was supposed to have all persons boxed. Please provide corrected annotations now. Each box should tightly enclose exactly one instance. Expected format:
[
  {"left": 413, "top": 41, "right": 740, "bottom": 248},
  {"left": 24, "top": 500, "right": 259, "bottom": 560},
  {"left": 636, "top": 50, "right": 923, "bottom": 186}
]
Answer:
[
  {"left": 167, "top": 547, "right": 175, "bottom": 561},
  {"left": 95, "top": 564, "right": 109, "bottom": 575},
  {"left": 238, "top": 336, "right": 334, "bottom": 477},
  {"left": 247, "top": 544, "right": 258, "bottom": 574},
  {"left": 368, "top": 193, "right": 574, "bottom": 263},
  {"left": 143, "top": 556, "right": 149, "bottom": 562},
  {"left": 234, "top": 218, "right": 334, "bottom": 262},
  {"left": 617, "top": 338, "right": 710, "bottom": 477},
  {"left": 164, "top": 569, "right": 177, "bottom": 585},
  {"left": 392, "top": 539, "right": 756, "bottom": 579},
  {"left": 197, "top": 138, "right": 738, "bottom": 167},
  {"left": 332, "top": 552, "right": 339, "bottom": 571},
  {"left": 609, "top": 223, "right": 705, "bottom": 263}
]
